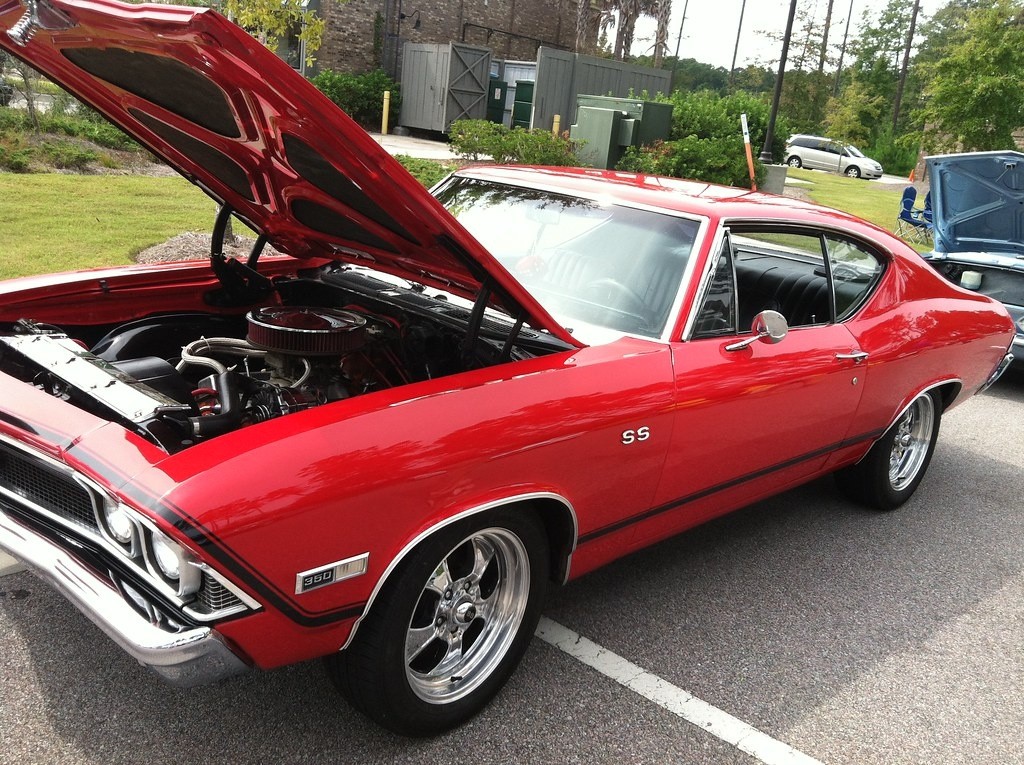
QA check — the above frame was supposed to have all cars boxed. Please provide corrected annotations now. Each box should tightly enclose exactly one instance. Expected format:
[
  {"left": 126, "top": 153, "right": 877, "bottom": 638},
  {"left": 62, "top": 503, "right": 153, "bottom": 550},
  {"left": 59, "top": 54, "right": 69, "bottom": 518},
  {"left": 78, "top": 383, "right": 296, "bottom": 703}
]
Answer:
[
  {"left": 899, "top": 148, "right": 1024, "bottom": 365},
  {"left": 0, "top": 0, "right": 1017, "bottom": 742}
]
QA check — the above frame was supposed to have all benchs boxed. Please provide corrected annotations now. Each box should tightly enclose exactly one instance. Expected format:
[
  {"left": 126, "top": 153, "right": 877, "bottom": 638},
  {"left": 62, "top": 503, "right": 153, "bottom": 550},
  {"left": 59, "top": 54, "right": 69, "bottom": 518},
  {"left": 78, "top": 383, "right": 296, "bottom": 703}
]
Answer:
[{"left": 546, "top": 248, "right": 866, "bottom": 336}]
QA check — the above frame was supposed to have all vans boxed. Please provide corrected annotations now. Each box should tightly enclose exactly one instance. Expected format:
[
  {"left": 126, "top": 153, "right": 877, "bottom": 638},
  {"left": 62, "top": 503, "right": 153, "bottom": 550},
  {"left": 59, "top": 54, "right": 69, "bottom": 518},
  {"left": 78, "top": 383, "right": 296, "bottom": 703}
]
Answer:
[{"left": 781, "top": 132, "right": 884, "bottom": 180}]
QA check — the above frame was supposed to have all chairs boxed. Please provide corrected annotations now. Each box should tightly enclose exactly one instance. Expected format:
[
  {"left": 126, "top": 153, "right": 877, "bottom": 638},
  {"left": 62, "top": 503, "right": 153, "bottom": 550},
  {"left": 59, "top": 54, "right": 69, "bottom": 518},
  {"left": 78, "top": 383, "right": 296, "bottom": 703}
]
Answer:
[{"left": 893, "top": 186, "right": 934, "bottom": 245}]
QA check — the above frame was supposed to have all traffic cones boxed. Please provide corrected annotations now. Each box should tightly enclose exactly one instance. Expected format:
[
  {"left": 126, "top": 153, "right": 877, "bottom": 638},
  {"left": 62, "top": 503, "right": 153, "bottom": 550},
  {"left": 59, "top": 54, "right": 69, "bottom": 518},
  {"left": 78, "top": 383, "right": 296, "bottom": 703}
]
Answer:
[{"left": 908, "top": 170, "right": 915, "bottom": 181}]
